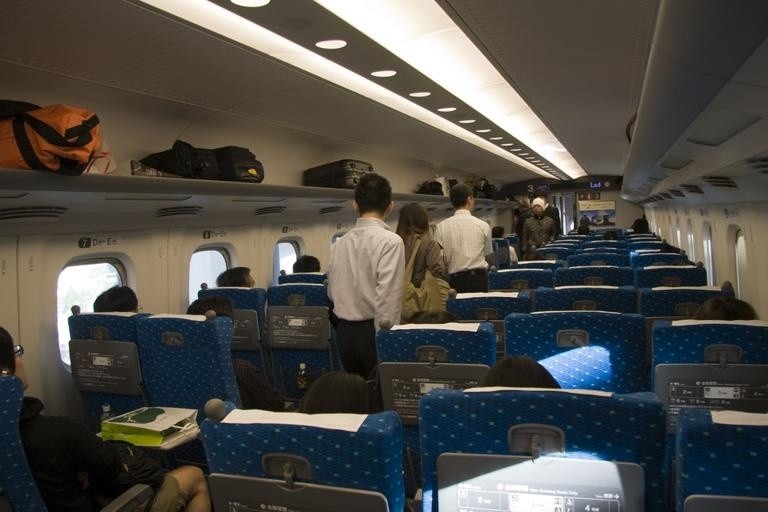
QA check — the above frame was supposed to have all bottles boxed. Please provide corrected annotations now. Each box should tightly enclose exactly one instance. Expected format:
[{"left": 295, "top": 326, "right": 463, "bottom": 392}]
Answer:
[
  {"left": 99, "top": 403, "right": 121, "bottom": 440},
  {"left": 297, "top": 362, "right": 311, "bottom": 395}
]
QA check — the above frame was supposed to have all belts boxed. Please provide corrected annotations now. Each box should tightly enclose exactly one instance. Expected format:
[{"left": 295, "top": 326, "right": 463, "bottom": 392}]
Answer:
[{"left": 451, "top": 268, "right": 484, "bottom": 277}]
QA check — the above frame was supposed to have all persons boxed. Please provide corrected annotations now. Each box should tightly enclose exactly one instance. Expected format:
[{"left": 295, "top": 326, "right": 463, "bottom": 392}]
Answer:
[
  {"left": 327, "top": 174, "right": 406, "bottom": 382},
  {"left": 534, "top": 190, "right": 560, "bottom": 240},
  {"left": 492, "top": 225, "right": 518, "bottom": 261},
  {"left": 0, "top": 327, "right": 212, "bottom": 512},
  {"left": 410, "top": 311, "right": 459, "bottom": 324},
  {"left": 603, "top": 230, "right": 617, "bottom": 240},
  {"left": 516, "top": 196, "right": 533, "bottom": 240},
  {"left": 186, "top": 297, "right": 286, "bottom": 411},
  {"left": 578, "top": 223, "right": 590, "bottom": 234},
  {"left": 293, "top": 255, "right": 321, "bottom": 273},
  {"left": 695, "top": 297, "right": 758, "bottom": 321},
  {"left": 436, "top": 183, "right": 496, "bottom": 294},
  {"left": 395, "top": 203, "right": 446, "bottom": 284},
  {"left": 299, "top": 370, "right": 378, "bottom": 411},
  {"left": 217, "top": 267, "right": 256, "bottom": 288},
  {"left": 93, "top": 283, "right": 143, "bottom": 313},
  {"left": 520, "top": 197, "right": 554, "bottom": 254},
  {"left": 483, "top": 353, "right": 561, "bottom": 388},
  {"left": 633, "top": 218, "right": 648, "bottom": 233}
]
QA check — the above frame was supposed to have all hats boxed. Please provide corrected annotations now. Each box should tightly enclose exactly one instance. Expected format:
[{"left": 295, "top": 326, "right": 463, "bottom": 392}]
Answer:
[
  {"left": 534, "top": 186, "right": 550, "bottom": 196},
  {"left": 532, "top": 198, "right": 545, "bottom": 210}
]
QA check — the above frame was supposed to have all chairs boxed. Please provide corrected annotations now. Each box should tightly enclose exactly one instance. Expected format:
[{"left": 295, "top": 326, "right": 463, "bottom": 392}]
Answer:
[{"left": 0, "top": 229, "right": 768, "bottom": 511}]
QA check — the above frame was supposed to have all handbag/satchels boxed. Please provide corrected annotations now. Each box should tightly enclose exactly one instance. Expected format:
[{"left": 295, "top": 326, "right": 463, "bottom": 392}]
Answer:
[
  {"left": 101, "top": 407, "right": 199, "bottom": 448},
  {"left": 400, "top": 276, "right": 451, "bottom": 324},
  {"left": 416, "top": 176, "right": 499, "bottom": 200},
  {"left": 0, "top": 103, "right": 102, "bottom": 176}
]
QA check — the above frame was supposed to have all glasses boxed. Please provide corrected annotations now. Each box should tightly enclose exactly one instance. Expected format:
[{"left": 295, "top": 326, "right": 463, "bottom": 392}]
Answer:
[{"left": 9, "top": 345, "right": 24, "bottom": 360}]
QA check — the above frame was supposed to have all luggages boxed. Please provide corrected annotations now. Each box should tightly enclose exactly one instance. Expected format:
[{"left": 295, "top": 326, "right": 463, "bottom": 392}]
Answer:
[
  {"left": 137, "top": 140, "right": 265, "bottom": 181},
  {"left": 303, "top": 159, "right": 373, "bottom": 188}
]
